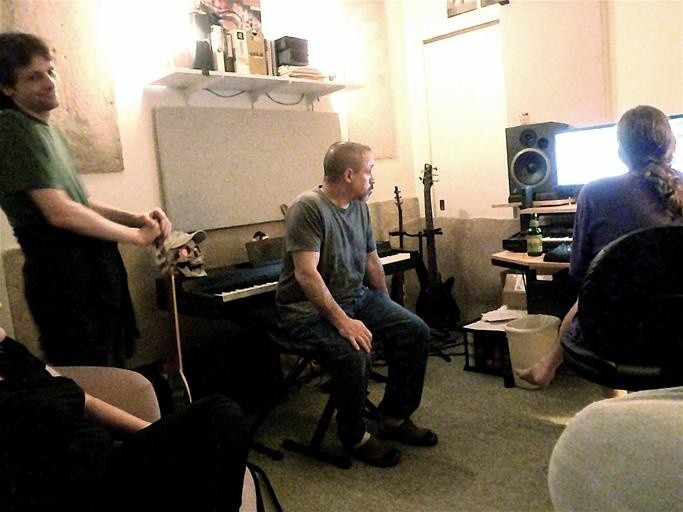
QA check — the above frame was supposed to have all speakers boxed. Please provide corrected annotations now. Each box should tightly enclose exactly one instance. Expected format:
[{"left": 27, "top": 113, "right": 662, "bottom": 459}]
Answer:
[{"left": 506, "top": 122, "right": 569, "bottom": 201}]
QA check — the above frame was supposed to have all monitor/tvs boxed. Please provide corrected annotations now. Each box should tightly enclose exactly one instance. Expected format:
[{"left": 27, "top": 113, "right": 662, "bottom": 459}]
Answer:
[{"left": 552, "top": 114, "right": 683, "bottom": 199}]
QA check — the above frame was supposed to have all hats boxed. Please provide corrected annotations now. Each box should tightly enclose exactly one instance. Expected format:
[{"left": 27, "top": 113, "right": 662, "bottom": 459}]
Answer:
[{"left": 162, "top": 230, "right": 206, "bottom": 249}]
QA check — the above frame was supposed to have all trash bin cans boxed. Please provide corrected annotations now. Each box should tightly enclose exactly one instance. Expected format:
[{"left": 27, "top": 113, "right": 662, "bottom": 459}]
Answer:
[{"left": 504, "top": 314, "right": 561, "bottom": 390}]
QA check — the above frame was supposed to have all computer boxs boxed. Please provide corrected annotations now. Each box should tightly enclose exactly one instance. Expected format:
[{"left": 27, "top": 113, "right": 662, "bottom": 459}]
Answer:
[{"left": 526, "top": 267, "right": 581, "bottom": 322}]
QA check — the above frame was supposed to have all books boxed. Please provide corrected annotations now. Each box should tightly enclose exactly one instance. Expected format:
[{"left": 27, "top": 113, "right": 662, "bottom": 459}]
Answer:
[{"left": 207, "top": 24, "right": 327, "bottom": 81}]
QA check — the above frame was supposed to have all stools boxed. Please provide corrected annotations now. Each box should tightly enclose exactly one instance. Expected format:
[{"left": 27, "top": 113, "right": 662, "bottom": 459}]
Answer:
[{"left": 261, "top": 317, "right": 377, "bottom": 470}]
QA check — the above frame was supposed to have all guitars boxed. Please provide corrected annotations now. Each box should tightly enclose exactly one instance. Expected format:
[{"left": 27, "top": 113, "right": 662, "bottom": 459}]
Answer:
[
  {"left": 415, "top": 163, "right": 460, "bottom": 330},
  {"left": 148, "top": 237, "right": 193, "bottom": 413},
  {"left": 503, "top": 221, "right": 575, "bottom": 253},
  {"left": 390, "top": 186, "right": 407, "bottom": 309}
]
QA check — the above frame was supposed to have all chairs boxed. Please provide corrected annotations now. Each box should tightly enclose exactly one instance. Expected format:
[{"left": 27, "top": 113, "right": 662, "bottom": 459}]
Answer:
[
  {"left": 560, "top": 223, "right": 683, "bottom": 388},
  {"left": 54, "top": 366, "right": 263, "bottom": 511}
]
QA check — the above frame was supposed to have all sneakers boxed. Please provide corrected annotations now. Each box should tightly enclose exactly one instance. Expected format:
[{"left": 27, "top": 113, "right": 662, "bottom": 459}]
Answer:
[
  {"left": 378, "top": 417, "right": 438, "bottom": 448},
  {"left": 349, "top": 431, "right": 402, "bottom": 467}
]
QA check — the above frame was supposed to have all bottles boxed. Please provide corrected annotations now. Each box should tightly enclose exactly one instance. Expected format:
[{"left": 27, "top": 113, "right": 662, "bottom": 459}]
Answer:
[{"left": 524, "top": 209, "right": 545, "bottom": 257}]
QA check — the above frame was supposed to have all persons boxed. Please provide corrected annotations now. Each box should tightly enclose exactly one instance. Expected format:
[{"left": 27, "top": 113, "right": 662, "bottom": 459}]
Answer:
[
  {"left": 1, "top": 327, "right": 251, "bottom": 511},
  {"left": 272, "top": 137, "right": 441, "bottom": 469},
  {"left": 508, "top": 104, "right": 682, "bottom": 397},
  {"left": 0, "top": 32, "right": 172, "bottom": 370}
]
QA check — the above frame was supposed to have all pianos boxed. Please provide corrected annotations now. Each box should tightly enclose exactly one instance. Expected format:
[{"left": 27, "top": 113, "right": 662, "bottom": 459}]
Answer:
[{"left": 155, "top": 240, "right": 420, "bottom": 321}]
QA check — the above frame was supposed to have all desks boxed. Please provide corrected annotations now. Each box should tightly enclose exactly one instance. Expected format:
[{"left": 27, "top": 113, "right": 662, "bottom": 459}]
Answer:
[{"left": 491, "top": 247, "right": 572, "bottom": 318}]
